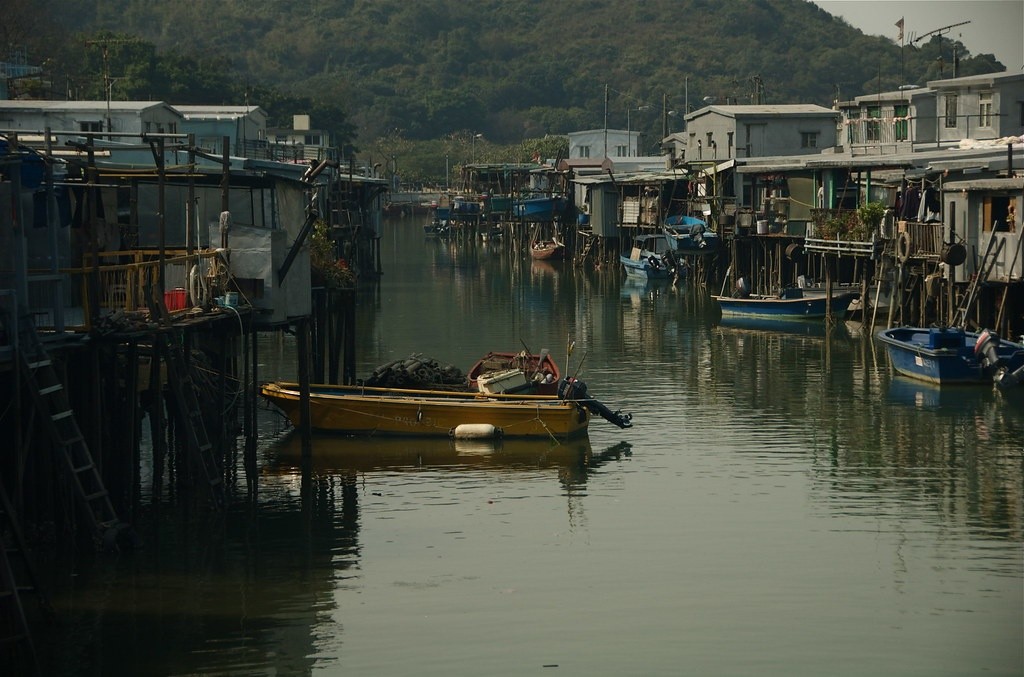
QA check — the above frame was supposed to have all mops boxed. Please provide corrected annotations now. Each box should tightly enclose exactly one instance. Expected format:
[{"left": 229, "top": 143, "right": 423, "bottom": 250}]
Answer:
[{"left": 219, "top": 210, "right": 231, "bottom": 248}]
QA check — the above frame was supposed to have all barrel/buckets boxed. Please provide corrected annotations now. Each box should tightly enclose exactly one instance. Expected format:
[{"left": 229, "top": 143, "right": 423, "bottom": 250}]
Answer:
[
  {"left": 578, "top": 213, "right": 589, "bottom": 225},
  {"left": 164, "top": 289, "right": 187, "bottom": 311},
  {"left": 756, "top": 220, "right": 767, "bottom": 235},
  {"left": 225, "top": 292, "right": 238, "bottom": 307}
]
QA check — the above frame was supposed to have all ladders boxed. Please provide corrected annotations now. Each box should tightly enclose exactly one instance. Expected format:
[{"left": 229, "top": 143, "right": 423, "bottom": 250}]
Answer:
[
  {"left": 0, "top": 301, "right": 124, "bottom": 552},
  {"left": 946, "top": 235, "right": 1007, "bottom": 328},
  {"left": 142, "top": 281, "right": 225, "bottom": 513}
]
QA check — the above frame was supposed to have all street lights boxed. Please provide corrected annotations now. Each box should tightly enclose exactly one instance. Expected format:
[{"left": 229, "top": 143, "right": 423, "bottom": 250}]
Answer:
[
  {"left": 472, "top": 133, "right": 483, "bottom": 173},
  {"left": 628, "top": 105, "right": 650, "bottom": 158}
]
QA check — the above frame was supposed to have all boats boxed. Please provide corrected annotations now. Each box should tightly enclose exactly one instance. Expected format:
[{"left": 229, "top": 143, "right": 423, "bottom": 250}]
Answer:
[
  {"left": 715, "top": 277, "right": 861, "bottom": 324},
  {"left": 876, "top": 322, "right": 1024, "bottom": 406},
  {"left": 529, "top": 239, "right": 564, "bottom": 261},
  {"left": 263, "top": 429, "right": 635, "bottom": 477},
  {"left": 625, "top": 275, "right": 680, "bottom": 292},
  {"left": 467, "top": 351, "right": 562, "bottom": 397},
  {"left": 261, "top": 380, "right": 633, "bottom": 438},
  {"left": 665, "top": 214, "right": 718, "bottom": 252},
  {"left": 421, "top": 195, "right": 516, "bottom": 236},
  {"left": 721, "top": 310, "right": 863, "bottom": 342},
  {"left": 889, "top": 373, "right": 1005, "bottom": 408},
  {"left": 513, "top": 195, "right": 569, "bottom": 220},
  {"left": 620, "top": 246, "right": 684, "bottom": 280}
]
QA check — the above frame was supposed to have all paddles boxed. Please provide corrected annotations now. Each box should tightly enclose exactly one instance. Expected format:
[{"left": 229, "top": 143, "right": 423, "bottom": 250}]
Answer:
[{"left": 531, "top": 348, "right": 550, "bottom": 379}]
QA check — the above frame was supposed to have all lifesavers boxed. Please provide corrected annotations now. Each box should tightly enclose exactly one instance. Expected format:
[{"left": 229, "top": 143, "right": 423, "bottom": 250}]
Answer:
[
  {"left": 896, "top": 231, "right": 912, "bottom": 263},
  {"left": 187, "top": 263, "right": 208, "bottom": 306}
]
